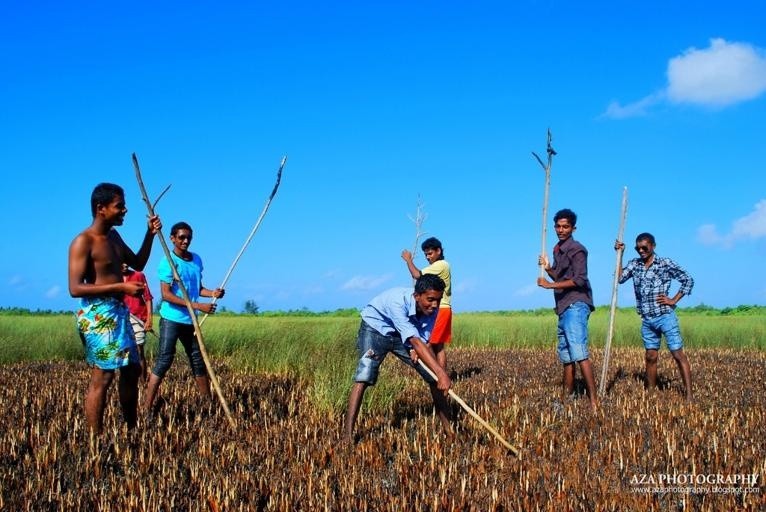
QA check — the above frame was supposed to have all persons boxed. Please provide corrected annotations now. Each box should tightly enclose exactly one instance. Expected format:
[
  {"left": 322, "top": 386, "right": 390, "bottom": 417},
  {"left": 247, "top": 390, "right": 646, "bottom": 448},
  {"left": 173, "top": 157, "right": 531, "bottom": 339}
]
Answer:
[
  {"left": 141, "top": 220, "right": 224, "bottom": 415},
  {"left": 68, "top": 182, "right": 163, "bottom": 436},
  {"left": 399, "top": 235, "right": 453, "bottom": 373},
  {"left": 339, "top": 272, "right": 457, "bottom": 446},
  {"left": 536, "top": 207, "right": 599, "bottom": 414},
  {"left": 613, "top": 231, "right": 695, "bottom": 401},
  {"left": 121, "top": 263, "right": 155, "bottom": 387}
]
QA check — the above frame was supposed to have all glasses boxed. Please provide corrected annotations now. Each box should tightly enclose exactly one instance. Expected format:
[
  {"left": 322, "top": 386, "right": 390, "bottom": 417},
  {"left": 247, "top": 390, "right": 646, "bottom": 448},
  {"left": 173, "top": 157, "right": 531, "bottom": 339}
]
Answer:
[
  {"left": 174, "top": 235, "right": 192, "bottom": 240},
  {"left": 635, "top": 246, "right": 649, "bottom": 251}
]
